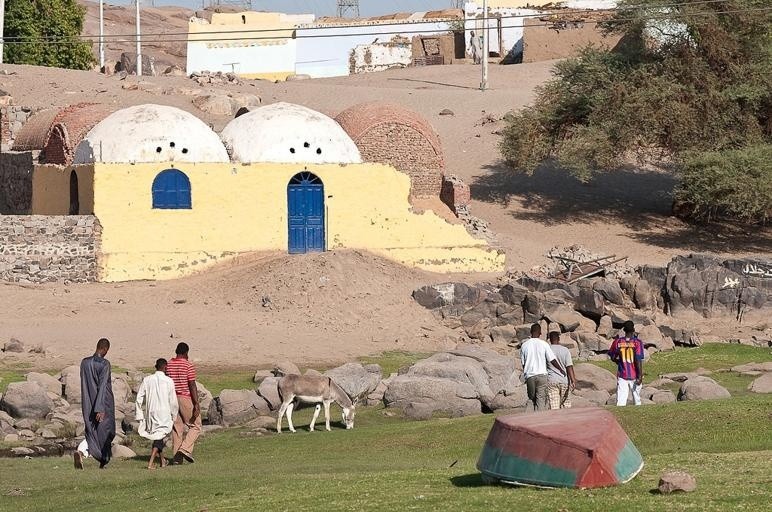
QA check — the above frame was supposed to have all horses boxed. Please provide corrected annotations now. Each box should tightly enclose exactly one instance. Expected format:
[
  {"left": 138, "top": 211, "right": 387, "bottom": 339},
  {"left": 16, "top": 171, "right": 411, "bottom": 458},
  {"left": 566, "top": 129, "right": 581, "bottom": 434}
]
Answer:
[{"left": 276, "top": 374, "right": 357, "bottom": 434}]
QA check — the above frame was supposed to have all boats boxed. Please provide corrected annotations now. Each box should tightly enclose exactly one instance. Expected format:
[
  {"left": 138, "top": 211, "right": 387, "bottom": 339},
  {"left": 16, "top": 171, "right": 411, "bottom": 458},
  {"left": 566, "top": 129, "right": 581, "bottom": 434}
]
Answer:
[{"left": 478, "top": 407, "right": 646, "bottom": 493}]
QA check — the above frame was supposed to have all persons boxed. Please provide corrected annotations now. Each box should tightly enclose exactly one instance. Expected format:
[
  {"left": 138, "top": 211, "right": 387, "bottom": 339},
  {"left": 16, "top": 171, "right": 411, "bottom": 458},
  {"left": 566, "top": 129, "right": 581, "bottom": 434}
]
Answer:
[
  {"left": 467, "top": 29, "right": 483, "bottom": 62},
  {"left": 164, "top": 342, "right": 202, "bottom": 465},
  {"left": 608, "top": 320, "right": 644, "bottom": 407},
  {"left": 73, "top": 337, "right": 116, "bottom": 471},
  {"left": 136, "top": 357, "right": 179, "bottom": 470},
  {"left": 520, "top": 324, "right": 566, "bottom": 411},
  {"left": 544, "top": 331, "right": 576, "bottom": 410}
]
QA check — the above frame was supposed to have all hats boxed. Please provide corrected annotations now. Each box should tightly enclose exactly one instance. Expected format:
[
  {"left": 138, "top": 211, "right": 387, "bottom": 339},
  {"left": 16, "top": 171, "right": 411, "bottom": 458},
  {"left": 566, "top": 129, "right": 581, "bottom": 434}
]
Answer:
[{"left": 623, "top": 321, "right": 636, "bottom": 332}]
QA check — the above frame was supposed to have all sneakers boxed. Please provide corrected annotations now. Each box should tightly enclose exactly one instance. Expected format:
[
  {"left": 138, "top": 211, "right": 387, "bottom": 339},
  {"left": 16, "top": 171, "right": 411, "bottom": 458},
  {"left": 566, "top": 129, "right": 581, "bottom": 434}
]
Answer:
[
  {"left": 74, "top": 452, "right": 84, "bottom": 470},
  {"left": 148, "top": 459, "right": 169, "bottom": 470},
  {"left": 173, "top": 449, "right": 194, "bottom": 464}
]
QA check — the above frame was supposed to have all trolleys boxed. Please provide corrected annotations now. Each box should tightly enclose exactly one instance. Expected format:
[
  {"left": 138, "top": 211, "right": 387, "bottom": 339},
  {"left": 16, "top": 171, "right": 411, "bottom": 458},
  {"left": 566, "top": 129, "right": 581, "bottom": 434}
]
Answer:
[{"left": 549, "top": 252, "right": 630, "bottom": 286}]
